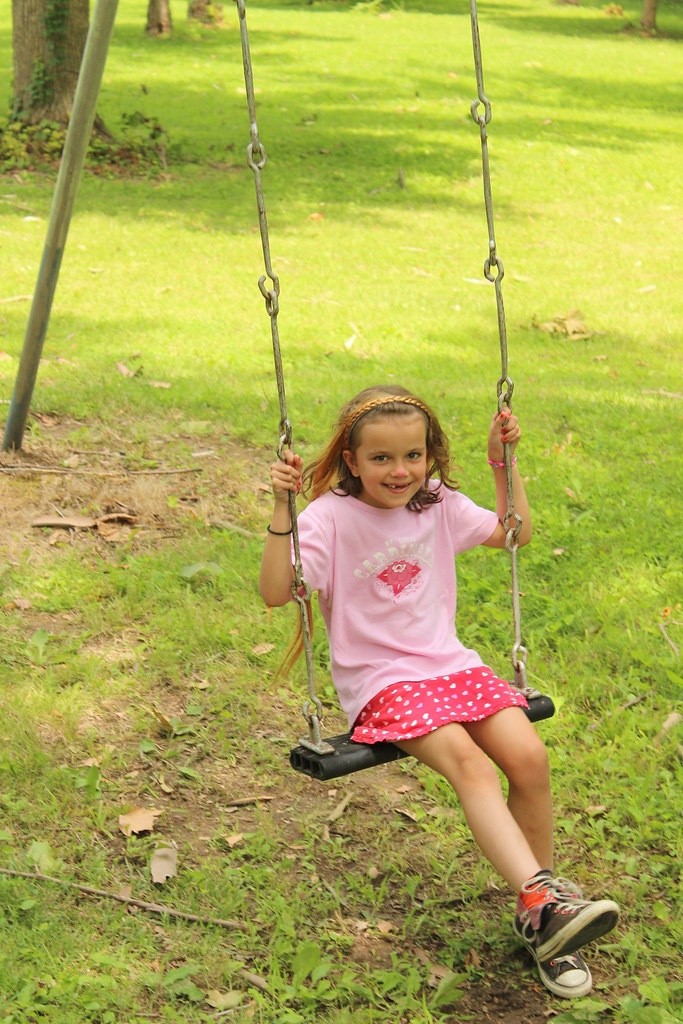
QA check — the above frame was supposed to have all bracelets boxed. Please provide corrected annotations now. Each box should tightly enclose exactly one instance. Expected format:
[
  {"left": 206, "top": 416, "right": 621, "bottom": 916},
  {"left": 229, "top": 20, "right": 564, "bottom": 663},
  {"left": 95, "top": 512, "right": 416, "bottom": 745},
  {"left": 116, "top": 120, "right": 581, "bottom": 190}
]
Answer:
[
  {"left": 489, "top": 457, "right": 516, "bottom": 469},
  {"left": 267, "top": 524, "right": 293, "bottom": 535}
]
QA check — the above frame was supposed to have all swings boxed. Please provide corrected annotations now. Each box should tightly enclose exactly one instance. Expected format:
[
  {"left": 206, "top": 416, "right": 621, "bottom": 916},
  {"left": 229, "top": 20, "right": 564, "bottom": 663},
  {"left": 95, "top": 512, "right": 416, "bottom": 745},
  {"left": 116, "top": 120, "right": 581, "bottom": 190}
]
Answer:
[{"left": 236, "top": 1, "right": 555, "bottom": 783}]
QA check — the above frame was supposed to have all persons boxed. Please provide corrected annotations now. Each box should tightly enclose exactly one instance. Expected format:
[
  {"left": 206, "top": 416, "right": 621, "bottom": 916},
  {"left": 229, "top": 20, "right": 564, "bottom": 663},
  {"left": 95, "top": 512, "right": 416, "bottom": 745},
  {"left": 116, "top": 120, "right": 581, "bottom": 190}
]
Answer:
[{"left": 260, "top": 385, "right": 621, "bottom": 1000}]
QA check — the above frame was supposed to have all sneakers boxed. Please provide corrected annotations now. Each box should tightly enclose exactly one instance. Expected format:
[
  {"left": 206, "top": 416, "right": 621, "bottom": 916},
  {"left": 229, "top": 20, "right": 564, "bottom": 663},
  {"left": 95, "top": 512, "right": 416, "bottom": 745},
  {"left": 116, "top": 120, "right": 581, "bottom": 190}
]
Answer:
[
  {"left": 515, "top": 870, "right": 618, "bottom": 965},
  {"left": 525, "top": 930, "right": 593, "bottom": 1000}
]
